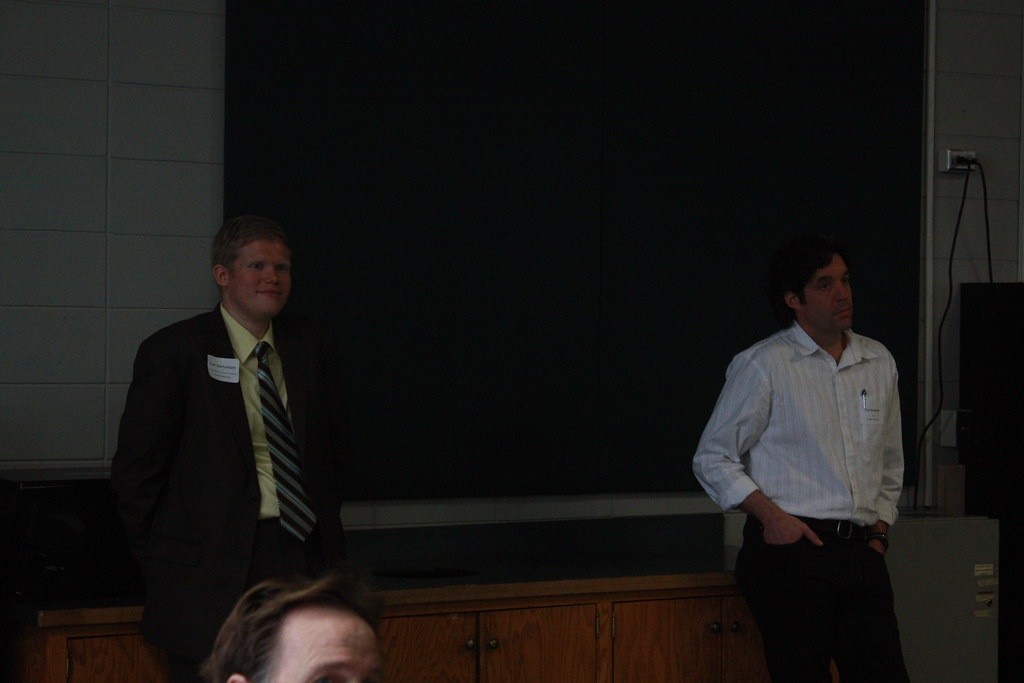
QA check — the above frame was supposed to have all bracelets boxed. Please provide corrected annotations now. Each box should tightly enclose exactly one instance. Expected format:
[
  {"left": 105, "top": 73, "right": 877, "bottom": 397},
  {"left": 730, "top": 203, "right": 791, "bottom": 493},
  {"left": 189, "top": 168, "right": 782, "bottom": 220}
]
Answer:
[{"left": 869, "top": 531, "right": 888, "bottom": 551}]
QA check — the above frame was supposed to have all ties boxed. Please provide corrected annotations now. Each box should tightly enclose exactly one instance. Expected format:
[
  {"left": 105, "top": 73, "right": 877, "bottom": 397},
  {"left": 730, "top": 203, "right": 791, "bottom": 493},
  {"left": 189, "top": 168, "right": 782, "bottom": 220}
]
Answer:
[{"left": 256, "top": 341, "right": 315, "bottom": 542}]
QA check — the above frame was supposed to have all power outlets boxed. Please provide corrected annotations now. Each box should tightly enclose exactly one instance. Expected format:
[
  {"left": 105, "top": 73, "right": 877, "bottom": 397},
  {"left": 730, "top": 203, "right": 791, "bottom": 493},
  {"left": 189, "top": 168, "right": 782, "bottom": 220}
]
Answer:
[{"left": 938, "top": 147, "right": 978, "bottom": 175}]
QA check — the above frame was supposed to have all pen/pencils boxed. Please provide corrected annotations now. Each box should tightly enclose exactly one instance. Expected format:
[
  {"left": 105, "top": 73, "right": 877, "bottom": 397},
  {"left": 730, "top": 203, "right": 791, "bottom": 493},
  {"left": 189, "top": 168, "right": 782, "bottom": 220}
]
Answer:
[{"left": 861, "top": 389, "right": 867, "bottom": 410}]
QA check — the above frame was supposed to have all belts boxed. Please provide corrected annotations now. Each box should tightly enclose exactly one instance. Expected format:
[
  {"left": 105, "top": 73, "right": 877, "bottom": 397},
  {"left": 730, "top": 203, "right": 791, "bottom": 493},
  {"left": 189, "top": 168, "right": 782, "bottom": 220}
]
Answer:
[{"left": 795, "top": 513, "right": 874, "bottom": 544}]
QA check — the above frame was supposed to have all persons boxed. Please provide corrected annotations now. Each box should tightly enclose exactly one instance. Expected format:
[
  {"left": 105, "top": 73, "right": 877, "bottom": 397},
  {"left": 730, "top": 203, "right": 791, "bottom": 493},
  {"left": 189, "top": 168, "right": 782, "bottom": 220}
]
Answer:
[
  {"left": 106, "top": 215, "right": 346, "bottom": 683},
  {"left": 693, "top": 230, "right": 910, "bottom": 683},
  {"left": 202, "top": 569, "right": 381, "bottom": 683}
]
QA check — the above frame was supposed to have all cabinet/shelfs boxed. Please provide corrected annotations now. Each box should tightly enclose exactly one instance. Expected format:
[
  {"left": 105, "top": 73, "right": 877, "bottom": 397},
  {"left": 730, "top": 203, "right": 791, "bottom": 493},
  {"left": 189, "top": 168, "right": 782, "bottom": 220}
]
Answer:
[
  {"left": 605, "top": 571, "right": 840, "bottom": 683},
  {"left": 358, "top": 577, "right": 606, "bottom": 683},
  {"left": 15, "top": 606, "right": 183, "bottom": 683}
]
